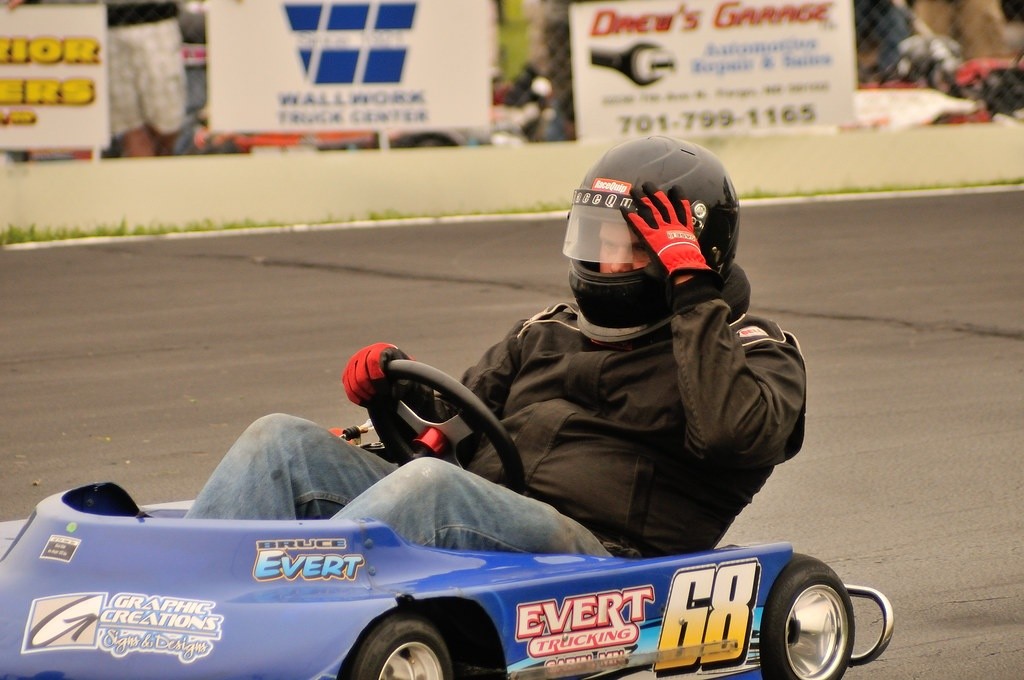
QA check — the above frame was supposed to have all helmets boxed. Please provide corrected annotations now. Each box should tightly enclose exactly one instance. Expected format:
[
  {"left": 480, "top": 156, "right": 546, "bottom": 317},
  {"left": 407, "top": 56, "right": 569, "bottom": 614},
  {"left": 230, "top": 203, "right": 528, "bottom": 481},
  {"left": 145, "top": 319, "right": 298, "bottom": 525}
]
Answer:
[{"left": 564, "top": 135, "right": 740, "bottom": 328}]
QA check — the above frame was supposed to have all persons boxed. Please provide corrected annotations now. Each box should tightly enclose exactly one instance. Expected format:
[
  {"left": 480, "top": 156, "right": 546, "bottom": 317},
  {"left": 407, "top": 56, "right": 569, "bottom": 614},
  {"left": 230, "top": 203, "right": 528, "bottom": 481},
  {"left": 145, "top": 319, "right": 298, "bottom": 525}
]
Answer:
[
  {"left": 507, "top": 21, "right": 578, "bottom": 145},
  {"left": 182, "top": 138, "right": 809, "bottom": 559},
  {"left": 104, "top": 0, "right": 187, "bottom": 159}
]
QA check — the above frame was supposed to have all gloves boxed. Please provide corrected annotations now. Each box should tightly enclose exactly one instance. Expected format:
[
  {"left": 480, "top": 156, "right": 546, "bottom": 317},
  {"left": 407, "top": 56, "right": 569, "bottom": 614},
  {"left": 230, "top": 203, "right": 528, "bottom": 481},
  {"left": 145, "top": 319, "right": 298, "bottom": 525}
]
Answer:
[
  {"left": 619, "top": 182, "right": 715, "bottom": 274},
  {"left": 342, "top": 343, "right": 415, "bottom": 409}
]
